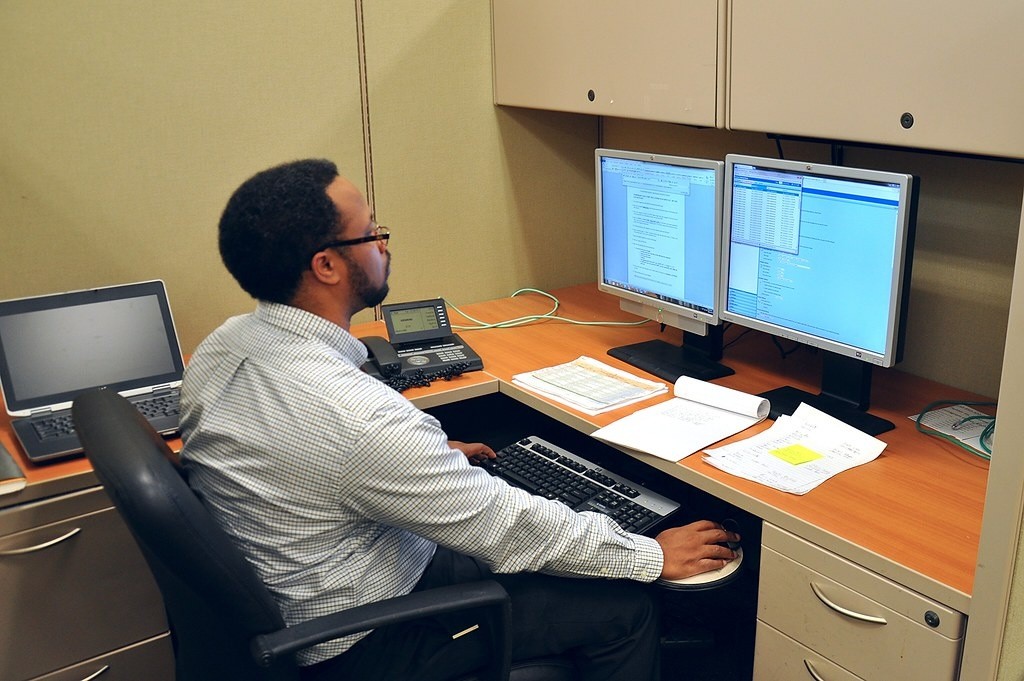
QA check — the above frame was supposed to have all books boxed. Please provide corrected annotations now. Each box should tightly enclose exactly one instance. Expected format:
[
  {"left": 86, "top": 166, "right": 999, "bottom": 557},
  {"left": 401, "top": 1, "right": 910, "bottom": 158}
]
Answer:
[{"left": 0, "top": 440, "right": 27, "bottom": 496}]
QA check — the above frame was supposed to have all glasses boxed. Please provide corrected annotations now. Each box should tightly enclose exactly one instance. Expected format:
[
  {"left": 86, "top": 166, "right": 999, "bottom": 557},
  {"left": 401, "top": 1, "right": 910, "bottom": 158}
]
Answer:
[{"left": 324, "top": 220, "right": 391, "bottom": 248}]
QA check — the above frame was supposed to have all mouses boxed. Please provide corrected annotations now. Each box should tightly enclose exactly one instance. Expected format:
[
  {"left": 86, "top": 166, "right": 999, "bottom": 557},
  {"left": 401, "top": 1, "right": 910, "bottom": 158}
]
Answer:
[{"left": 712, "top": 541, "right": 740, "bottom": 552}]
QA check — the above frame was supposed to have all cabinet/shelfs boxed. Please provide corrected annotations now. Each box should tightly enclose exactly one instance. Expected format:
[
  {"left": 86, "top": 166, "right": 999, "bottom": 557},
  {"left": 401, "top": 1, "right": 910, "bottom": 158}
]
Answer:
[
  {"left": 752, "top": 522, "right": 965, "bottom": 681},
  {"left": 0, "top": 491, "right": 176, "bottom": 681}
]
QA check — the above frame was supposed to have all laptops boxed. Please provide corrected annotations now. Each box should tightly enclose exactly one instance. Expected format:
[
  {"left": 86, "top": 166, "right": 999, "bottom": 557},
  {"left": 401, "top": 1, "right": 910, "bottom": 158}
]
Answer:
[{"left": 0, "top": 278, "right": 186, "bottom": 467}]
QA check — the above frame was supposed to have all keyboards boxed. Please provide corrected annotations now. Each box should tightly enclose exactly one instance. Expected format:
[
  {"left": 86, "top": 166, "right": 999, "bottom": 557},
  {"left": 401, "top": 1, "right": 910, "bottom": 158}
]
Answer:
[{"left": 476, "top": 435, "right": 682, "bottom": 536}]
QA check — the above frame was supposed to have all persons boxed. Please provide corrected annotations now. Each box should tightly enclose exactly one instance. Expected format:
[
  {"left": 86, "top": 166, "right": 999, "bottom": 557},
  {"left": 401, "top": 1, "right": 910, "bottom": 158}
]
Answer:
[{"left": 177, "top": 158, "right": 740, "bottom": 681}]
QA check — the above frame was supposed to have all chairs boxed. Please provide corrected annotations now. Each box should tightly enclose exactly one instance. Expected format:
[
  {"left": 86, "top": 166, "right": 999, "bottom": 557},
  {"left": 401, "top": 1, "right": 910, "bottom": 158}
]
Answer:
[{"left": 68, "top": 384, "right": 514, "bottom": 681}]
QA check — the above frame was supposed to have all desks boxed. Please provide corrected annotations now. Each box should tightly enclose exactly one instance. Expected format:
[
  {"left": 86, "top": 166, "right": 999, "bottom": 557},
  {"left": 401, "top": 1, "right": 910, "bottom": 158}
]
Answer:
[
  {"left": 0, "top": 319, "right": 499, "bottom": 508},
  {"left": 382, "top": 282, "right": 998, "bottom": 616}
]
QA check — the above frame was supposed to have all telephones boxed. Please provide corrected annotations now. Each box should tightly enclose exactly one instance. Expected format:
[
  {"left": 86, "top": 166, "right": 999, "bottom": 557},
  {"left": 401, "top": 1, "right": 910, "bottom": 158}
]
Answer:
[{"left": 354, "top": 299, "right": 485, "bottom": 383}]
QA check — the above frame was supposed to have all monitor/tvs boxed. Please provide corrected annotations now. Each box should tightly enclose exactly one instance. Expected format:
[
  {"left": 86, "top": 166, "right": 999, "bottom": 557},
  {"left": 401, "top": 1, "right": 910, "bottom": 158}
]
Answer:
[
  {"left": 596, "top": 148, "right": 735, "bottom": 384},
  {"left": 719, "top": 153, "right": 921, "bottom": 434}
]
